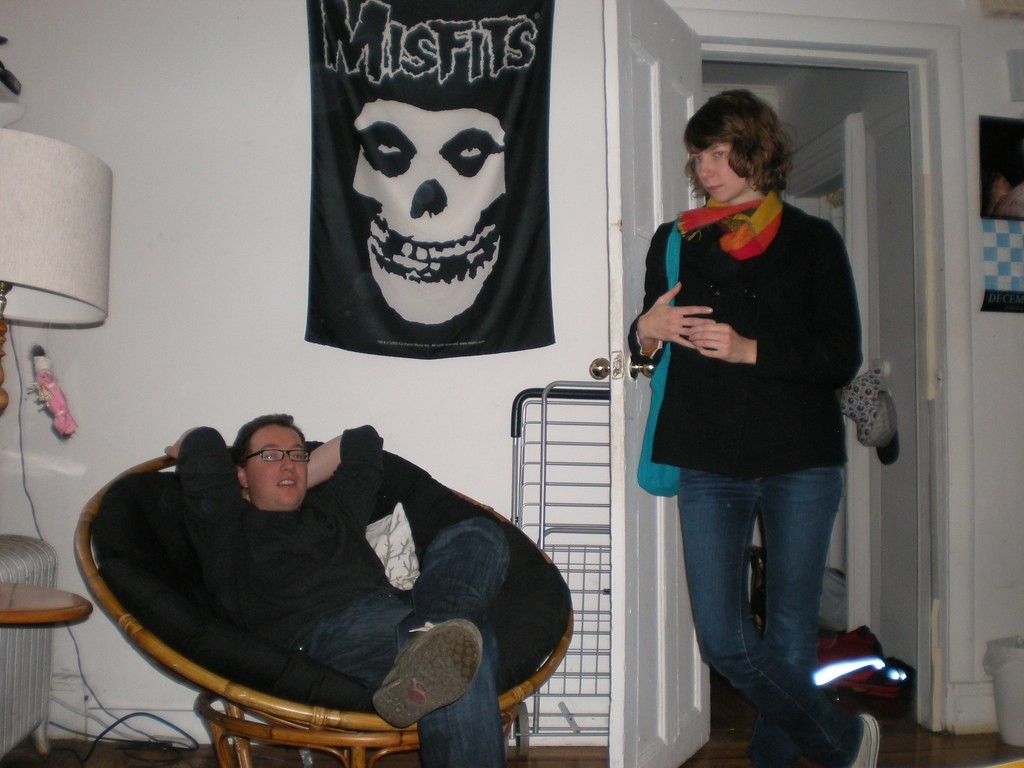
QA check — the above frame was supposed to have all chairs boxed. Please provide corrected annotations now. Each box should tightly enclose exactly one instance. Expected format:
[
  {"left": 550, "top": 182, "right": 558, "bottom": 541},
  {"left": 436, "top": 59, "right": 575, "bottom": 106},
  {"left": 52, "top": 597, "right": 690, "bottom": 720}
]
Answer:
[{"left": 72, "top": 452, "right": 574, "bottom": 768}]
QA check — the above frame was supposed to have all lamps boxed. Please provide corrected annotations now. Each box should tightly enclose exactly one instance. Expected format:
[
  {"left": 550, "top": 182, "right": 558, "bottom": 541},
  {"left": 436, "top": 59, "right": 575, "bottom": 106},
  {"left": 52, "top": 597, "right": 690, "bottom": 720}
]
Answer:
[{"left": 0, "top": 126, "right": 113, "bottom": 409}]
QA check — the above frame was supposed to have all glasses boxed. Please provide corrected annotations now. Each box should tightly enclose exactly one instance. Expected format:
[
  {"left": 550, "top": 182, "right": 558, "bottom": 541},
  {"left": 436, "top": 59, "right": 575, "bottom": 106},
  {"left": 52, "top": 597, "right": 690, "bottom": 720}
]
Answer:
[{"left": 239, "top": 449, "right": 310, "bottom": 463}]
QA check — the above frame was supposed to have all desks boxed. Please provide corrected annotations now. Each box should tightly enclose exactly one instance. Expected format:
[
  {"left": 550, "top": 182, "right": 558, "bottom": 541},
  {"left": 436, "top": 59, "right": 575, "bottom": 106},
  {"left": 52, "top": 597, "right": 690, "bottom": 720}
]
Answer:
[{"left": 0, "top": 581, "right": 92, "bottom": 624}]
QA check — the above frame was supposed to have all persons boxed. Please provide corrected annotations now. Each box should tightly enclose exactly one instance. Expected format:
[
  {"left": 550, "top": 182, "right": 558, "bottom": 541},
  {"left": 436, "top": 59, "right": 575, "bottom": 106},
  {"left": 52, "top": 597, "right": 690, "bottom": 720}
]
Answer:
[
  {"left": 628, "top": 90, "right": 882, "bottom": 768},
  {"left": 166, "top": 415, "right": 512, "bottom": 768}
]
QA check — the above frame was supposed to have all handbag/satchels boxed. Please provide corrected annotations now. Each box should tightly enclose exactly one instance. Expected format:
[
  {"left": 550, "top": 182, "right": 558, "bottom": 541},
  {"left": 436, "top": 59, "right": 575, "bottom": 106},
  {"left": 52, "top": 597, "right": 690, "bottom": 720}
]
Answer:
[
  {"left": 817, "top": 625, "right": 884, "bottom": 687},
  {"left": 639, "top": 211, "right": 684, "bottom": 496}
]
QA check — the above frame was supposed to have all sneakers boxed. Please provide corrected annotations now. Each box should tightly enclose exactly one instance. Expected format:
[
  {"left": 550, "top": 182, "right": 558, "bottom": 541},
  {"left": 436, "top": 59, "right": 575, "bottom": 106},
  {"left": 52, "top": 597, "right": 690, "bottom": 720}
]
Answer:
[{"left": 849, "top": 712, "right": 880, "bottom": 768}]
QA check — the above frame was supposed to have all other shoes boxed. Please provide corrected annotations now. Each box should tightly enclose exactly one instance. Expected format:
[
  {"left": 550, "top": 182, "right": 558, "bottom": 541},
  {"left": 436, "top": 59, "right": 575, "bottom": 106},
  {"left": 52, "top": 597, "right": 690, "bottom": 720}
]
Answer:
[{"left": 374, "top": 619, "right": 482, "bottom": 728}]
